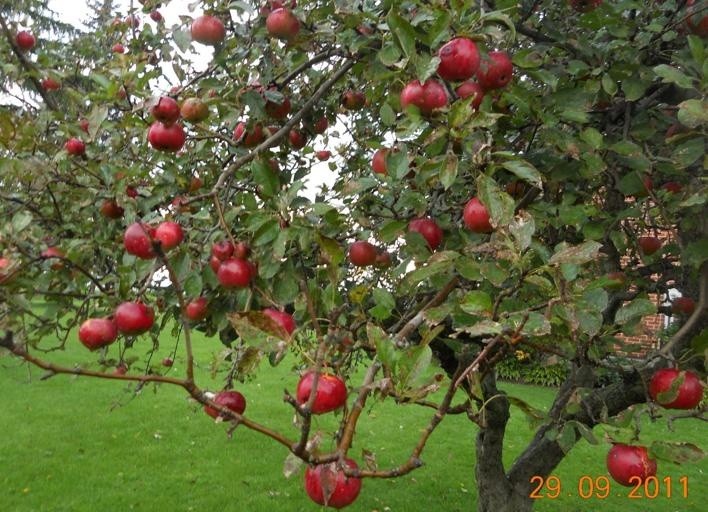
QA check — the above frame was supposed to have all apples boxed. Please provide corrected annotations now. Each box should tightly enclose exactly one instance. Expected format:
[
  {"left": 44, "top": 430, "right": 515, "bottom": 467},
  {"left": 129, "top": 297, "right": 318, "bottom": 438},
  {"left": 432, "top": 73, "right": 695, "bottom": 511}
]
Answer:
[
  {"left": 638, "top": 236, "right": 661, "bottom": 256},
  {"left": 408, "top": 218, "right": 443, "bottom": 250},
  {"left": 372, "top": 147, "right": 415, "bottom": 181},
  {"left": 209, "top": 240, "right": 259, "bottom": 289},
  {"left": 101, "top": 199, "right": 125, "bottom": 220},
  {"left": 401, "top": 80, "right": 446, "bottom": 119},
  {"left": 154, "top": 220, "right": 184, "bottom": 251},
  {"left": 671, "top": 296, "right": 696, "bottom": 316},
  {"left": 262, "top": 309, "right": 295, "bottom": 337},
  {"left": 149, "top": 121, "right": 185, "bottom": 153},
  {"left": 433, "top": 35, "right": 480, "bottom": 81},
  {"left": 234, "top": 1, "right": 367, "bottom": 198},
  {"left": 188, "top": 14, "right": 225, "bottom": 47},
  {"left": 16, "top": 31, "right": 35, "bottom": 52},
  {"left": 116, "top": 300, "right": 155, "bottom": 336},
  {"left": 455, "top": 78, "right": 484, "bottom": 110},
  {"left": 462, "top": 196, "right": 493, "bottom": 233},
  {"left": 296, "top": 370, "right": 348, "bottom": 415},
  {"left": 477, "top": 50, "right": 512, "bottom": 89},
  {"left": 650, "top": 367, "right": 704, "bottom": 410},
  {"left": 67, "top": 137, "right": 86, "bottom": 156},
  {"left": 79, "top": 119, "right": 91, "bottom": 132},
  {"left": 665, "top": 182, "right": 683, "bottom": 195},
  {"left": 205, "top": 390, "right": 246, "bottom": 421},
  {"left": 606, "top": 442, "right": 656, "bottom": 488},
  {"left": 107, "top": 11, "right": 163, "bottom": 100},
  {"left": 305, "top": 456, "right": 361, "bottom": 509},
  {"left": 505, "top": 179, "right": 525, "bottom": 199},
  {"left": 151, "top": 96, "right": 181, "bottom": 123},
  {"left": 124, "top": 222, "right": 157, "bottom": 261},
  {"left": 79, "top": 318, "right": 117, "bottom": 352},
  {"left": 40, "top": 76, "right": 60, "bottom": 91},
  {"left": 0, "top": 259, "right": 16, "bottom": 284},
  {"left": 183, "top": 96, "right": 210, "bottom": 124},
  {"left": 376, "top": 251, "right": 392, "bottom": 268},
  {"left": 349, "top": 240, "right": 376, "bottom": 267},
  {"left": 115, "top": 170, "right": 202, "bottom": 213},
  {"left": 328, "top": 329, "right": 355, "bottom": 353},
  {"left": 642, "top": 174, "right": 653, "bottom": 196},
  {"left": 186, "top": 297, "right": 209, "bottom": 320},
  {"left": 41, "top": 247, "right": 65, "bottom": 270}
]
